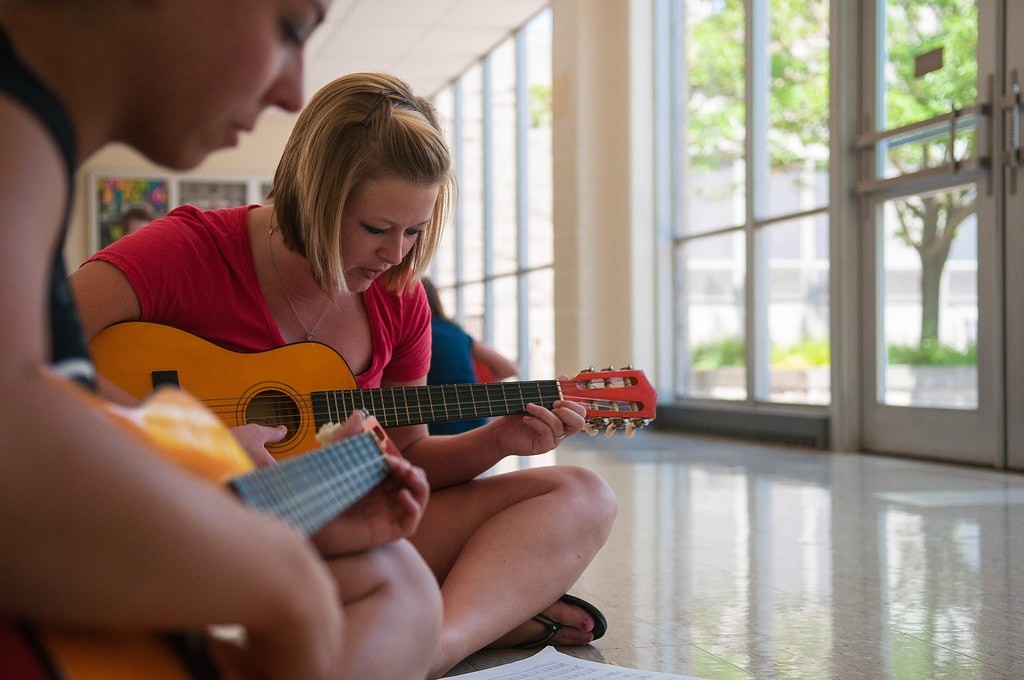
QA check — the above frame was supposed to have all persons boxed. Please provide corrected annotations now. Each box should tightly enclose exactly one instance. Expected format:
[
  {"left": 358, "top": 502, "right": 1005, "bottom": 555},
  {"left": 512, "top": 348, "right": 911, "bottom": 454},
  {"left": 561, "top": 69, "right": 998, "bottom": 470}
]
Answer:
[
  {"left": 0, "top": 0, "right": 445, "bottom": 680},
  {"left": 69, "top": 74, "right": 616, "bottom": 680}
]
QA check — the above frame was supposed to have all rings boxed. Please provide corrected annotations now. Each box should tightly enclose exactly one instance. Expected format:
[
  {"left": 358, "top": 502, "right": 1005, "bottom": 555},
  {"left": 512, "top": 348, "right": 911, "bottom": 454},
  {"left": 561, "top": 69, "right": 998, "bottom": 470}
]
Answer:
[{"left": 556, "top": 433, "right": 566, "bottom": 440}]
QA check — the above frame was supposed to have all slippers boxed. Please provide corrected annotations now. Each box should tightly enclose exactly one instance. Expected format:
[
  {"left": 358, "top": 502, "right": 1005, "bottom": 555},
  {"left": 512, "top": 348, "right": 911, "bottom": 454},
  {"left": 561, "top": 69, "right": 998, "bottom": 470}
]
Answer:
[{"left": 489, "top": 593, "right": 607, "bottom": 649}]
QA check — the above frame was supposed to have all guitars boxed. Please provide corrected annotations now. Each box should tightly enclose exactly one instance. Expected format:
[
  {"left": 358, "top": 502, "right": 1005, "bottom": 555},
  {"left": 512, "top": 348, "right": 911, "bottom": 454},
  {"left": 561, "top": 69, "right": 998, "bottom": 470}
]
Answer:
[
  {"left": 89, "top": 316, "right": 659, "bottom": 469},
  {"left": 0, "top": 382, "right": 390, "bottom": 680}
]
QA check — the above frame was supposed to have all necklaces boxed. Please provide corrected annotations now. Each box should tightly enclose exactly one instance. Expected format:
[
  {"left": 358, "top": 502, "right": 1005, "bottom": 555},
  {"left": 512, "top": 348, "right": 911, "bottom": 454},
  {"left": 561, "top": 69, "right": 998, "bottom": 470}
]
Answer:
[{"left": 271, "top": 213, "right": 331, "bottom": 338}]
